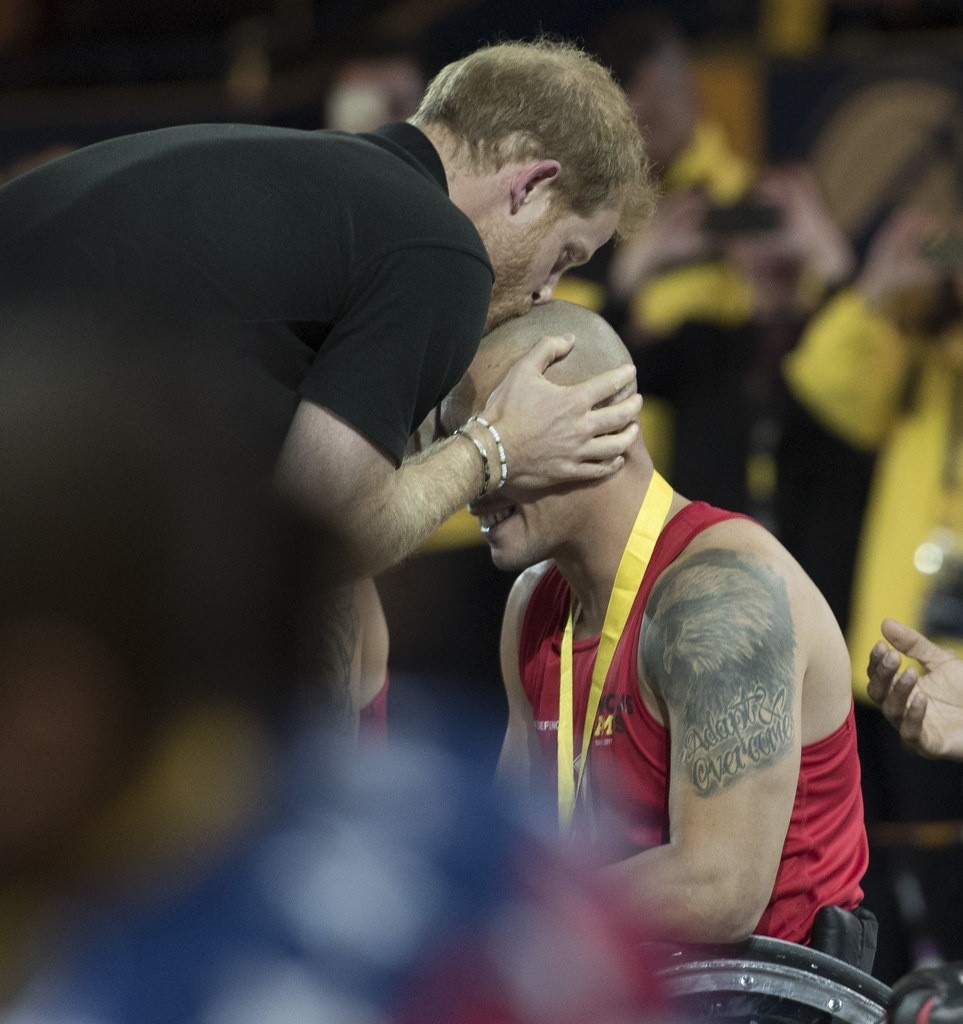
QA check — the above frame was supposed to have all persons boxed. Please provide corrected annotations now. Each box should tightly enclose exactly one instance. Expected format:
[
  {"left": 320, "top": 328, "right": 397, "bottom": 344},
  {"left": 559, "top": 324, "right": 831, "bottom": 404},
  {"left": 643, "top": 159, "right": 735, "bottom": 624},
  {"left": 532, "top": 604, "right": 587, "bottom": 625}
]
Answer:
[{"left": 2, "top": 0, "right": 962, "bottom": 1022}]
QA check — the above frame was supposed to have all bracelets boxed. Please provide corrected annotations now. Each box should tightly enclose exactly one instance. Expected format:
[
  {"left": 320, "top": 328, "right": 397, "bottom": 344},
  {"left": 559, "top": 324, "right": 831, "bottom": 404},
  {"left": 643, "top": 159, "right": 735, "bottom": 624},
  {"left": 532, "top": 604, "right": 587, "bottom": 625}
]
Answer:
[
  {"left": 467, "top": 414, "right": 509, "bottom": 490},
  {"left": 451, "top": 429, "right": 491, "bottom": 496}
]
